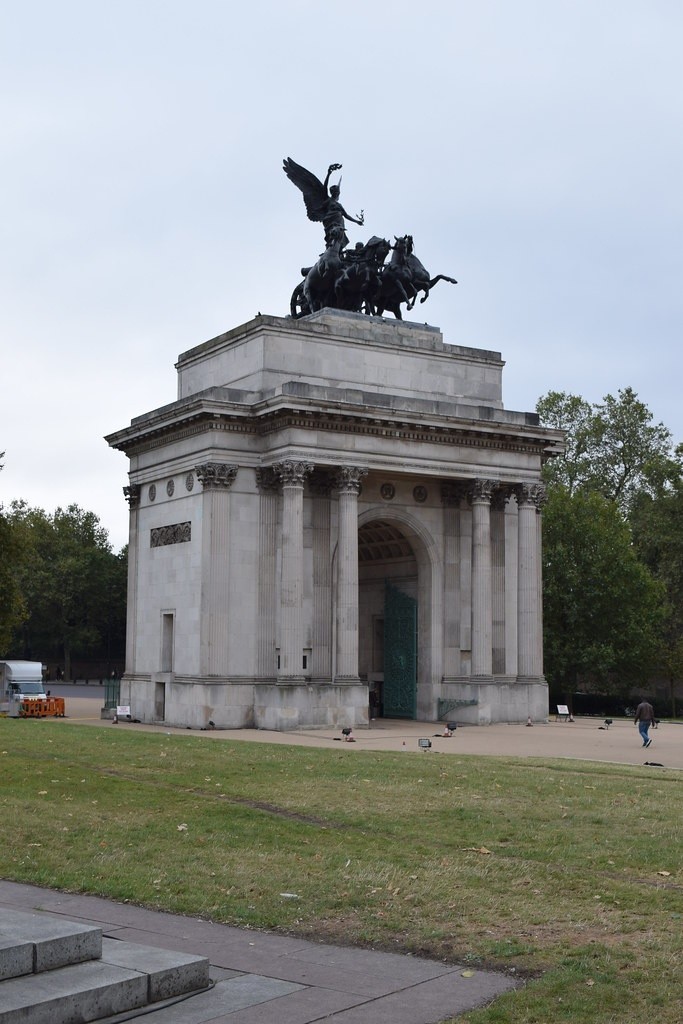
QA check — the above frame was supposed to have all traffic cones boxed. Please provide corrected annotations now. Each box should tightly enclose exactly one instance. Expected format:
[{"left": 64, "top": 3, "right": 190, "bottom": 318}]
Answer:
[
  {"left": 112, "top": 714, "right": 117, "bottom": 725},
  {"left": 442, "top": 726, "right": 451, "bottom": 737},
  {"left": 526, "top": 716, "right": 534, "bottom": 726},
  {"left": 568, "top": 713, "right": 575, "bottom": 722}
]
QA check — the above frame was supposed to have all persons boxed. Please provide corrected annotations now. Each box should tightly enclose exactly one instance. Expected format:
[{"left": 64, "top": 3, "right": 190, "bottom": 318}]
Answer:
[
  {"left": 369, "top": 688, "right": 377, "bottom": 721},
  {"left": 323, "top": 167, "right": 363, "bottom": 250},
  {"left": 634, "top": 695, "right": 655, "bottom": 748}
]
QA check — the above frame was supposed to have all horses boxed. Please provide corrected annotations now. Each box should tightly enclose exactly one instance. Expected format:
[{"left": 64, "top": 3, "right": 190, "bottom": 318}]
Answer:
[{"left": 302, "top": 221, "right": 459, "bottom": 321}]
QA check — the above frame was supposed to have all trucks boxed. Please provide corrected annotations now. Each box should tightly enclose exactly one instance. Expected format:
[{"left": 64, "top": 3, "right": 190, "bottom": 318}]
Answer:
[{"left": 0, "top": 660, "right": 50, "bottom": 707}]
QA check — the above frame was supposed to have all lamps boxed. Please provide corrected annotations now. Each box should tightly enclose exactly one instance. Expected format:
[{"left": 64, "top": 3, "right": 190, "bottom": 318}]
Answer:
[
  {"left": 127, "top": 715, "right": 131, "bottom": 719},
  {"left": 342, "top": 728, "right": 350, "bottom": 735},
  {"left": 655, "top": 719, "right": 661, "bottom": 725},
  {"left": 418, "top": 739, "right": 432, "bottom": 748},
  {"left": 447, "top": 722, "right": 457, "bottom": 730},
  {"left": 209, "top": 721, "right": 216, "bottom": 727},
  {"left": 604, "top": 718, "right": 613, "bottom": 724}
]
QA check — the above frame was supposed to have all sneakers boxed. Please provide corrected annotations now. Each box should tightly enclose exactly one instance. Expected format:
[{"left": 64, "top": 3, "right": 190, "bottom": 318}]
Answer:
[{"left": 643, "top": 739, "right": 652, "bottom": 748}]
